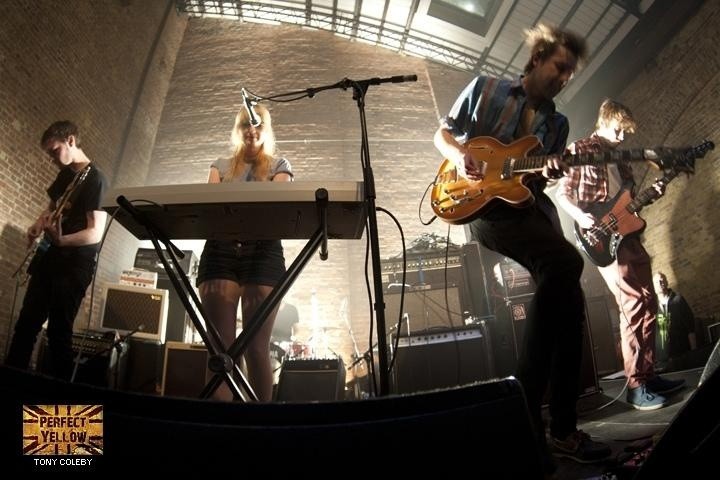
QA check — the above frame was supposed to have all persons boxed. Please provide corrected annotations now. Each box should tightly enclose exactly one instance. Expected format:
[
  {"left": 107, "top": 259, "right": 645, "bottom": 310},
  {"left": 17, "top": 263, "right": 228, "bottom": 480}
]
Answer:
[
  {"left": 430, "top": 21, "right": 616, "bottom": 467},
  {"left": 555, "top": 96, "right": 689, "bottom": 410},
  {"left": 648, "top": 271, "right": 697, "bottom": 374},
  {"left": 195, "top": 99, "right": 293, "bottom": 401},
  {"left": 4, "top": 118, "right": 111, "bottom": 383},
  {"left": 271, "top": 292, "right": 300, "bottom": 352}
]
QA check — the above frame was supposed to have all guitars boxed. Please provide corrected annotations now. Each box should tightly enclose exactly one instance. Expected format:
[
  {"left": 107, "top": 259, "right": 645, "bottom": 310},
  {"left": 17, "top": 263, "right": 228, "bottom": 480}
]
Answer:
[
  {"left": 573, "top": 139, "right": 714, "bottom": 267},
  {"left": 430, "top": 135, "right": 696, "bottom": 226},
  {"left": 15, "top": 165, "right": 91, "bottom": 283}
]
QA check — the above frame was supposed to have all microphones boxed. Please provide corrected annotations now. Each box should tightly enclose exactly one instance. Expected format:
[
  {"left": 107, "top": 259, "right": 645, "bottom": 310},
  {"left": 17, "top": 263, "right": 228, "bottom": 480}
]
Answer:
[{"left": 241, "top": 90, "right": 262, "bottom": 128}]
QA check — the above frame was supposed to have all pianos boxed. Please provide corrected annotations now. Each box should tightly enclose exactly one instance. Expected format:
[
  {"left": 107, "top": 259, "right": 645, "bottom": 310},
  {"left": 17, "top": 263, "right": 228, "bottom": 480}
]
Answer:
[{"left": 100, "top": 180, "right": 373, "bottom": 240}]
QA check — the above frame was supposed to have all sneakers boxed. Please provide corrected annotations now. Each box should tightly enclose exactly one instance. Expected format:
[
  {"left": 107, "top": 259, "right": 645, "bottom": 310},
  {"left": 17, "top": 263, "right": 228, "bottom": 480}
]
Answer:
[
  {"left": 548, "top": 430, "right": 612, "bottom": 463},
  {"left": 627, "top": 386, "right": 667, "bottom": 411},
  {"left": 646, "top": 377, "right": 686, "bottom": 395}
]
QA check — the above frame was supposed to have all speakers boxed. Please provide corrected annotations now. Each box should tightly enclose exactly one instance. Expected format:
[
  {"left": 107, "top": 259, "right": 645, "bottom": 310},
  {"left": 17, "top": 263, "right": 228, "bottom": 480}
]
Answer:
[
  {"left": 118, "top": 248, "right": 199, "bottom": 392},
  {"left": 273, "top": 356, "right": 346, "bottom": 402},
  {"left": 501, "top": 261, "right": 600, "bottom": 408},
  {"left": 97, "top": 281, "right": 169, "bottom": 346},
  {"left": 160, "top": 341, "right": 234, "bottom": 401},
  {"left": 382, "top": 284, "right": 466, "bottom": 345},
  {"left": 390, "top": 321, "right": 492, "bottom": 396}
]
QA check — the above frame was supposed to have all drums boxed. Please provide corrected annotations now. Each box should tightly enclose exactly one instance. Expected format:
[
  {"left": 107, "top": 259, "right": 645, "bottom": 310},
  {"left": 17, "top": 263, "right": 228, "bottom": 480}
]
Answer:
[{"left": 285, "top": 341, "right": 316, "bottom": 361}]
